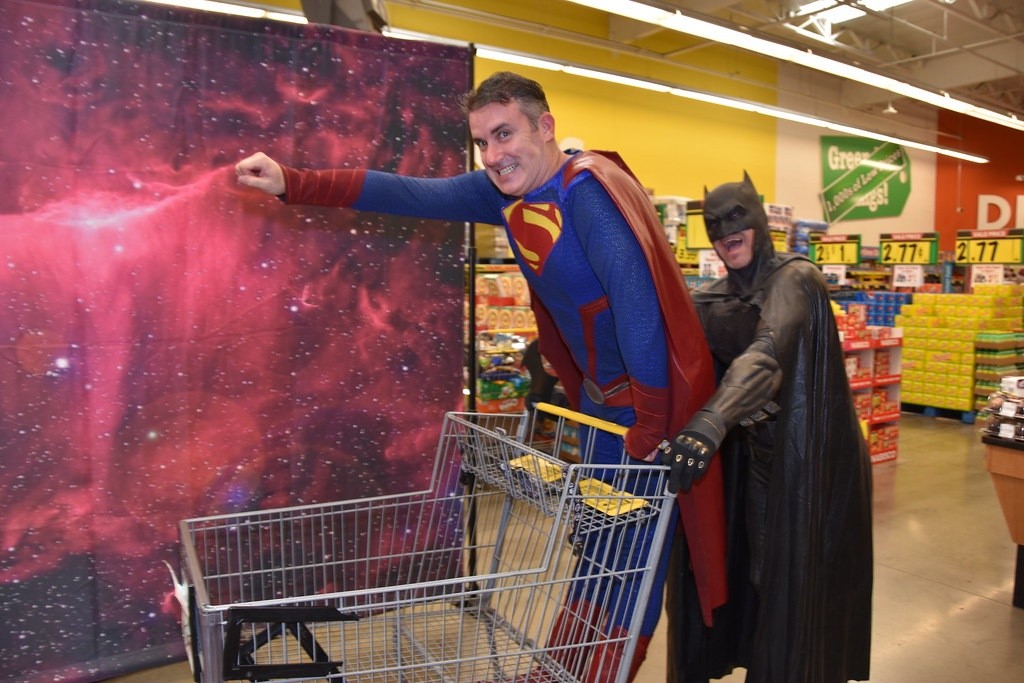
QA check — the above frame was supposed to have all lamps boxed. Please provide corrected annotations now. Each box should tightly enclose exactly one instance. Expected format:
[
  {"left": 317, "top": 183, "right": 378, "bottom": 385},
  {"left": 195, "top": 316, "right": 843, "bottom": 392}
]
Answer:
[
  {"left": 138, "top": 0, "right": 990, "bottom": 165},
  {"left": 570, "top": 0, "right": 1023, "bottom": 132}
]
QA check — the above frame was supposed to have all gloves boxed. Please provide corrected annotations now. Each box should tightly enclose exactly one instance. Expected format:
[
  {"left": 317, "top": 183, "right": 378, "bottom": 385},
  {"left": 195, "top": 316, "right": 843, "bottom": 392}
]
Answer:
[{"left": 661, "top": 432, "right": 715, "bottom": 494}]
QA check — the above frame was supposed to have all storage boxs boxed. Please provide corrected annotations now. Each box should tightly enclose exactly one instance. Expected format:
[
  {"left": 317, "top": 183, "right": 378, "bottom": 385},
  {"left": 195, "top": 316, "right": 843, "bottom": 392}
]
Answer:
[{"left": 470, "top": 201, "right": 1024, "bottom": 471}]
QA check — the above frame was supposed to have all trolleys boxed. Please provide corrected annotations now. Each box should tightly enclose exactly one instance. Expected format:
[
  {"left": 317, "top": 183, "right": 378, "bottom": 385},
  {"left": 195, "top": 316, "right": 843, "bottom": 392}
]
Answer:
[{"left": 181, "top": 396, "right": 679, "bottom": 683}]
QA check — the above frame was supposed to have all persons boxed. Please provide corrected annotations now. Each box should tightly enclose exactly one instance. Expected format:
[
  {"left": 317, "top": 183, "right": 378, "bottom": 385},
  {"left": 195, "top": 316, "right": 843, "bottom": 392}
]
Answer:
[
  {"left": 641, "top": 171, "right": 877, "bottom": 683},
  {"left": 234, "top": 71, "right": 726, "bottom": 683}
]
